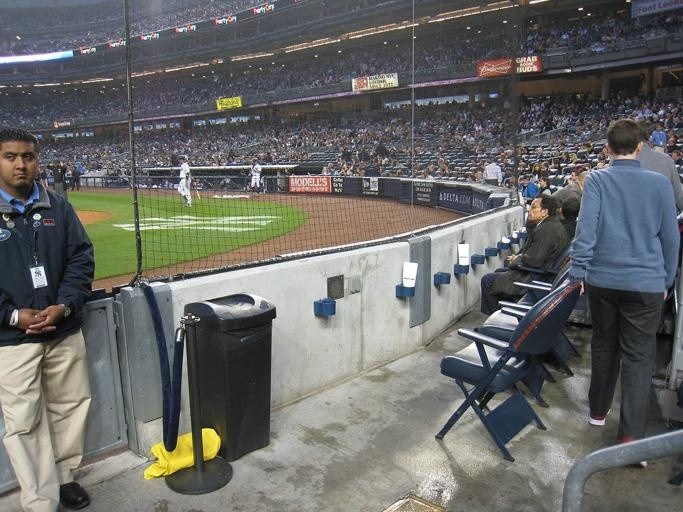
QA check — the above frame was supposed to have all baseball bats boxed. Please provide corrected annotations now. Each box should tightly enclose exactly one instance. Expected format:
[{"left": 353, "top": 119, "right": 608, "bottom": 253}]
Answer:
[{"left": 191, "top": 180, "right": 201, "bottom": 201}]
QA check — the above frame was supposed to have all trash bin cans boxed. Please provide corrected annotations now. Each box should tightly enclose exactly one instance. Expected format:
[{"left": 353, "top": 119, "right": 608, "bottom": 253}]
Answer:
[{"left": 184, "top": 292, "right": 277, "bottom": 463}]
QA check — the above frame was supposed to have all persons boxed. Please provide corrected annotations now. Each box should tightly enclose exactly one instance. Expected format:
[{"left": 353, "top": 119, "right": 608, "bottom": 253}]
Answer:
[
  {"left": 566, "top": 119, "right": 681, "bottom": 471},
  {"left": 479, "top": 193, "right": 568, "bottom": 318},
  {"left": 1, "top": 127, "right": 95, "bottom": 512},
  {"left": 0, "top": 1, "right": 682, "bottom": 240}
]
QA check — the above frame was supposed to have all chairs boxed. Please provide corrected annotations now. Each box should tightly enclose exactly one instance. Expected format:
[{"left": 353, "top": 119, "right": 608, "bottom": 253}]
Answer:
[
  {"left": 481, "top": 243, "right": 591, "bottom": 407},
  {"left": 293, "top": 110, "right": 683, "bottom": 204},
  {"left": 434, "top": 279, "right": 584, "bottom": 460}
]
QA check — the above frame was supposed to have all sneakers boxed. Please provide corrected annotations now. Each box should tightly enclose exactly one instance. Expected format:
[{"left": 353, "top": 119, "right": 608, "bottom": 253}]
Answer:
[
  {"left": 587, "top": 408, "right": 611, "bottom": 426},
  {"left": 60, "top": 481, "right": 90, "bottom": 511},
  {"left": 617, "top": 435, "right": 647, "bottom": 468}
]
